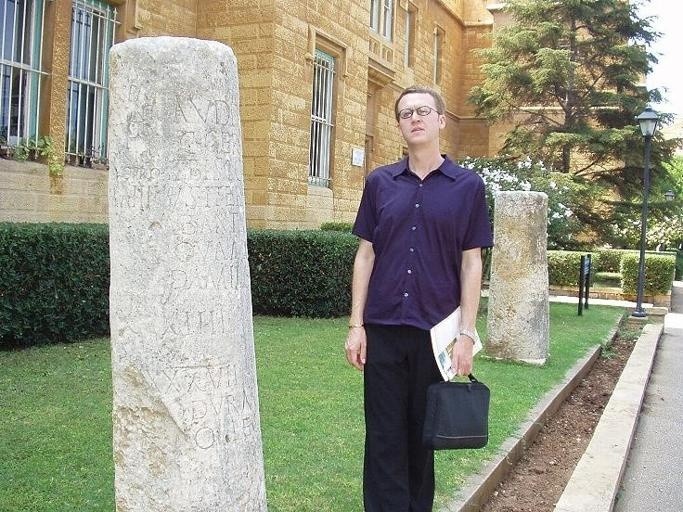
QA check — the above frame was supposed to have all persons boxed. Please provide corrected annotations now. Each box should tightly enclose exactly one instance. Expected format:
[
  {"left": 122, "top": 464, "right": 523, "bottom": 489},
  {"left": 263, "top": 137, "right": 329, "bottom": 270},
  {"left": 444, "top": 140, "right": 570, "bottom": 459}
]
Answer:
[{"left": 343, "top": 87, "right": 490, "bottom": 511}]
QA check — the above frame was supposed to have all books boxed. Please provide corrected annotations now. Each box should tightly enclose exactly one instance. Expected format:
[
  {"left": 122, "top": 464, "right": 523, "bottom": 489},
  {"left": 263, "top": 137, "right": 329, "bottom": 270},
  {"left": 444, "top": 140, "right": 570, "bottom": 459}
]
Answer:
[
  {"left": 348, "top": 324, "right": 364, "bottom": 329},
  {"left": 429, "top": 306, "right": 482, "bottom": 382}
]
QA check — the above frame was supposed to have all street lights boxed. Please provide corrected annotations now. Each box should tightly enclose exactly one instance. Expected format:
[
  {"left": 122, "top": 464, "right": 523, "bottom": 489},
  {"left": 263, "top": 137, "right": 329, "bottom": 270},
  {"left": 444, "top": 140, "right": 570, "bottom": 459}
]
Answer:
[{"left": 632, "top": 106, "right": 659, "bottom": 317}]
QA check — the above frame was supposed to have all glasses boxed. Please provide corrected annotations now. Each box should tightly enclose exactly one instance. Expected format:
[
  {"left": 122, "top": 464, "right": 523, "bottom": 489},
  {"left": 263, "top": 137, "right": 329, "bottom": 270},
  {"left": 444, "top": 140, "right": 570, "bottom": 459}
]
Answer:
[{"left": 397, "top": 105, "right": 440, "bottom": 124}]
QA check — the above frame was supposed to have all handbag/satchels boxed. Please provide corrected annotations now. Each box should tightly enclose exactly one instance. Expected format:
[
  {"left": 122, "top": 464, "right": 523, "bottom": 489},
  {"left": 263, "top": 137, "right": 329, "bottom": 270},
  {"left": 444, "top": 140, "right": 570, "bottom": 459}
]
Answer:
[{"left": 421, "top": 368, "right": 490, "bottom": 449}]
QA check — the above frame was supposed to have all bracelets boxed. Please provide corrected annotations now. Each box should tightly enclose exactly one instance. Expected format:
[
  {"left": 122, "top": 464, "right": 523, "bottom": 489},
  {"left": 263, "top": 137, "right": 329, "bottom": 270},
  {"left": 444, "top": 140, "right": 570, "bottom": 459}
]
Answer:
[{"left": 457, "top": 328, "right": 476, "bottom": 345}]
